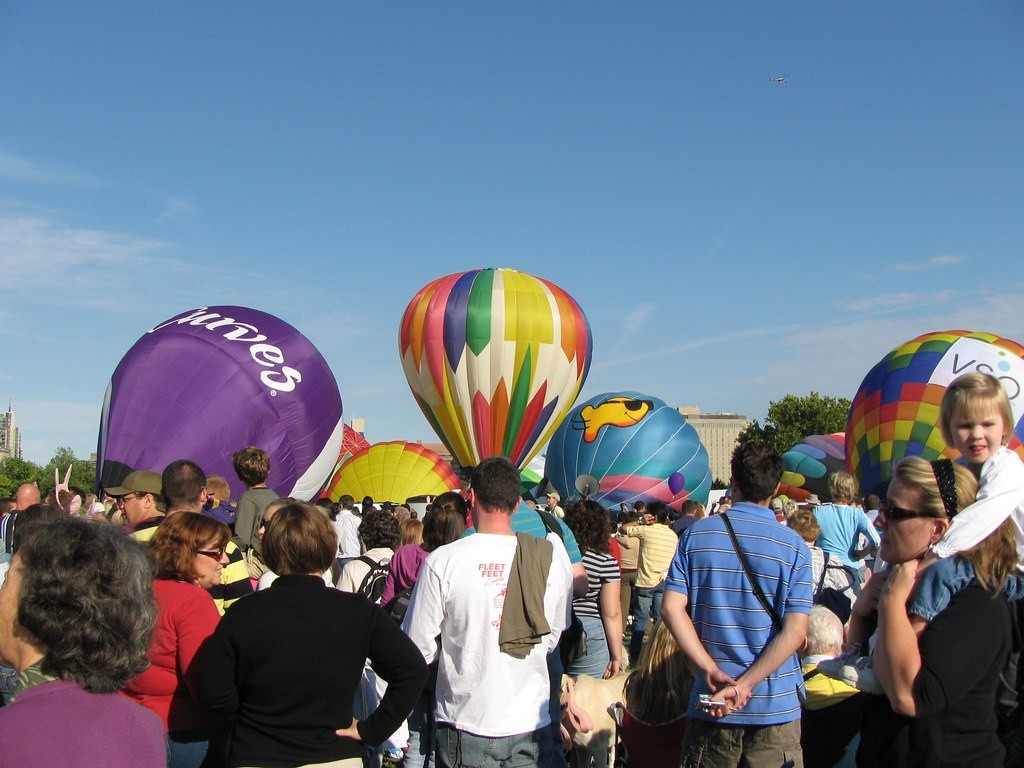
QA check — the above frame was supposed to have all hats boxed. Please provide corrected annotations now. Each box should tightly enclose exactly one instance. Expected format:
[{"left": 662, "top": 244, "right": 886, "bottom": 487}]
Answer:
[
  {"left": 546, "top": 493, "right": 560, "bottom": 502},
  {"left": 770, "top": 498, "right": 782, "bottom": 512},
  {"left": 104, "top": 470, "right": 162, "bottom": 498},
  {"left": 805, "top": 494, "right": 821, "bottom": 504}
]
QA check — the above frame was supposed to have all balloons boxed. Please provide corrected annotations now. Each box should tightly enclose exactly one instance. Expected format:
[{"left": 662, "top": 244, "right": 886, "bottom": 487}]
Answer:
[{"left": 669, "top": 473, "right": 685, "bottom": 494}]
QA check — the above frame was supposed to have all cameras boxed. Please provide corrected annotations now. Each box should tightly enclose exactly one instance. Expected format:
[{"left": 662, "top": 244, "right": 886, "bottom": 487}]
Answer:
[{"left": 694, "top": 693, "right": 726, "bottom": 712}]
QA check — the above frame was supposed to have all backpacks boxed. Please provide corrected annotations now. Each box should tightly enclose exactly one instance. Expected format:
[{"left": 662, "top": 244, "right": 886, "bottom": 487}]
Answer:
[{"left": 354, "top": 556, "right": 391, "bottom": 605}]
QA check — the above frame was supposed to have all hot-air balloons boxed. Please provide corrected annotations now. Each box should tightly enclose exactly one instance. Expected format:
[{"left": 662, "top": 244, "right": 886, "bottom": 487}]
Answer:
[
  {"left": 398, "top": 266, "right": 594, "bottom": 473},
  {"left": 844, "top": 328, "right": 1024, "bottom": 502},
  {"left": 310, "top": 424, "right": 373, "bottom": 507},
  {"left": 766, "top": 431, "right": 851, "bottom": 509},
  {"left": 520, "top": 392, "right": 713, "bottom": 524},
  {"left": 94, "top": 306, "right": 344, "bottom": 506},
  {"left": 326, "top": 441, "right": 463, "bottom": 503}
]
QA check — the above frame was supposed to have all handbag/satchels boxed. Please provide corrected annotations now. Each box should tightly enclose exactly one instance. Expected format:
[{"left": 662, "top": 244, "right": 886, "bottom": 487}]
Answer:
[{"left": 812, "top": 552, "right": 855, "bottom": 626}]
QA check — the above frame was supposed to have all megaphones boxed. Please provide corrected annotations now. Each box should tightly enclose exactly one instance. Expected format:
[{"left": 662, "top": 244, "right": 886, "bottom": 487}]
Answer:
[
  {"left": 575, "top": 475, "right": 600, "bottom": 500},
  {"left": 536, "top": 478, "right": 558, "bottom": 499}
]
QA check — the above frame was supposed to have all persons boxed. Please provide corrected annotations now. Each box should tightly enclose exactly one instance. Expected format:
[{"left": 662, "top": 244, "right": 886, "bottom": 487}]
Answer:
[
  {"left": 169, "top": 502, "right": 428, "bottom": 768},
  {"left": 662, "top": 444, "right": 813, "bottom": 768},
  {"left": 849, "top": 456, "right": 1009, "bottom": 768},
  {"left": 0, "top": 446, "right": 880, "bottom": 768},
  {"left": 402, "top": 455, "right": 565, "bottom": 768},
  {"left": 918, "top": 374, "right": 1024, "bottom": 767},
  {"left": 118, "top": 510, "right": 232, "bottom": 768},
  {"left": 0, "top": 515, "right": 168, "bottom": 768}
]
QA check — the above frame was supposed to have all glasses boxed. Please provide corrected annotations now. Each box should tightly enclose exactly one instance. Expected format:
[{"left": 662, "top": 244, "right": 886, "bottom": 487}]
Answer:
[
  {"left": 120, "top": 496, "right": 144, "bottom": 503},
  {"left": 884, "top": 505, "right": 937, "bottom": 520},
  {"left": 194, "top": 549, "right": 224, "bottom": 559},
  {"left": 260, "top": 517, "right": 269, "bottom": 529}
]
